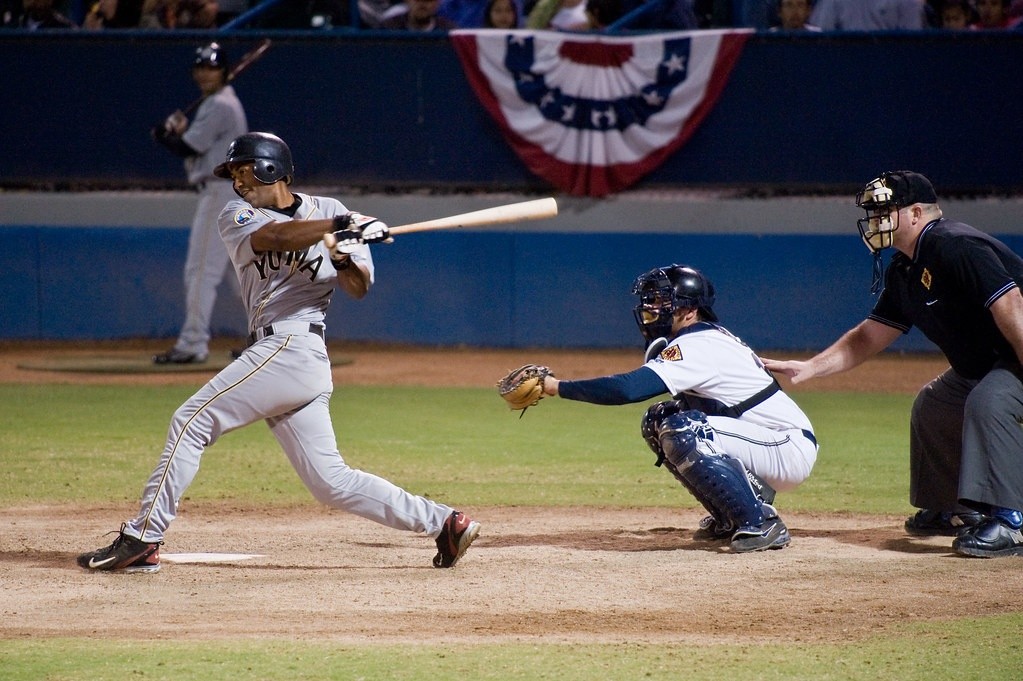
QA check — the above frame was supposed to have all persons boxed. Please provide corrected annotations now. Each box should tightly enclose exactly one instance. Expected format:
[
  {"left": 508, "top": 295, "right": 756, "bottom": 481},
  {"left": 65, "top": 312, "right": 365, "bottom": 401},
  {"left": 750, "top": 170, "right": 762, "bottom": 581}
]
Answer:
[
  {"left": 78, "top": 132, "right": 480, "bottom": 575},
  {"left": 0, "top": 0, "right": 1023, "bottom": 28},
  {"left": 496, "top": 264, "right": 819, "bottom": 553},
  {"left": 152, "top": 41, "right": 248, "bottom": 363},
  {"left": 758, "top": 171, "right": 1023, "bottom": 559}
]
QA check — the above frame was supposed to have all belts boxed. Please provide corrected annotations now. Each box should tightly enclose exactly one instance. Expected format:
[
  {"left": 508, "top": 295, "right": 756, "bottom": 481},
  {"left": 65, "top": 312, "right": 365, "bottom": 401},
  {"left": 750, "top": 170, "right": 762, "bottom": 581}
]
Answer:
[
  {"left": 243, "top": 321, "right": 323, "bottom": 342},
  {"left": 802, "top": 428, "right": 816, "bottom": 449}
]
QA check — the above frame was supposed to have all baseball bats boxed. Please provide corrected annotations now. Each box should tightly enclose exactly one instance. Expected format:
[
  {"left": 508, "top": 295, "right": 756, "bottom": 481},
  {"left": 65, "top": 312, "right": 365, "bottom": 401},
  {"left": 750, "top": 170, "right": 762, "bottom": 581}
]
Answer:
[
  {"left": 152, "top": 38, "right": 272, "bottom": 145},
  {"left": 324, "top": 198, "right": 558, "bottom": 248}
]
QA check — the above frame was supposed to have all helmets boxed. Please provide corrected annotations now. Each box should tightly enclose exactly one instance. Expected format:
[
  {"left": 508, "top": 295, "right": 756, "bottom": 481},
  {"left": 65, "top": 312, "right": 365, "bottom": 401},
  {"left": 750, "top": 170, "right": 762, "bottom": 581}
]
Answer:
[
  {"left": 630, "top": 264, "right": 719, "bottom": 343},
  {"left": 213, "top": 133, "right": 295, "bottom": 187},
  {"left": 186, "top": 39, "right": 235, "bottom": 85}
]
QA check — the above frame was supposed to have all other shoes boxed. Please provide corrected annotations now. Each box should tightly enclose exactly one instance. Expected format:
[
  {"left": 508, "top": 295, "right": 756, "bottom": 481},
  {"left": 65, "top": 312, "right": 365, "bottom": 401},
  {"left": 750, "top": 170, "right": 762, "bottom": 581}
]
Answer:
[{"left": 151, "top": 347, "right": 209, "bottom": 363}]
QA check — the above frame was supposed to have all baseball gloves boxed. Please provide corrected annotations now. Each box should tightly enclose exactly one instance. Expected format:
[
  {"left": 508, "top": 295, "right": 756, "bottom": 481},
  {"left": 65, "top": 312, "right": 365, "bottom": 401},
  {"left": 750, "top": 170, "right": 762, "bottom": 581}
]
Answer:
[{"left": 498, "top": 362, "right": 555, "bottom": 410}]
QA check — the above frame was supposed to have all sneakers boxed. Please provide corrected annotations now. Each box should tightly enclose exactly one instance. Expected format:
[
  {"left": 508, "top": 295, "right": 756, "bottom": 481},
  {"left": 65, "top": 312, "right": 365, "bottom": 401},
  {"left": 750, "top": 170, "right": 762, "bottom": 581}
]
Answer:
[
  {"left": 904, "top": 506, "right": 1023, "bottom": 558},
  {"left": 431, "top": 510, "right": 480, "bottom": 568},
  {"left": 694, "top": 515, "right": 792, "bottom": 553},
  {"left": 77, "top": 531, "right": 161, "bottom": 574}
]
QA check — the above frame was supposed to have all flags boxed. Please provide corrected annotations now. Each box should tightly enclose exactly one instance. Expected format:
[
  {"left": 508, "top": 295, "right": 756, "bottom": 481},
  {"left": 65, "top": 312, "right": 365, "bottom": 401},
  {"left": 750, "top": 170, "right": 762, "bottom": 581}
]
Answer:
[{"left": 449, "top": 27, "right": 756, "bottom": 196}]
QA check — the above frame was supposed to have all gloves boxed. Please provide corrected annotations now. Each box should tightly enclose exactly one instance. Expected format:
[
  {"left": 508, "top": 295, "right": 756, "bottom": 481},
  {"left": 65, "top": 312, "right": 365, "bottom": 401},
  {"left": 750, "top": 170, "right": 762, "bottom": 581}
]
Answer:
[
  {"left": 334, "top": 210, "right": 394, "bottom": 245},
  {"left": 324, "top": 228, "right": 366, "bottom": 271}
]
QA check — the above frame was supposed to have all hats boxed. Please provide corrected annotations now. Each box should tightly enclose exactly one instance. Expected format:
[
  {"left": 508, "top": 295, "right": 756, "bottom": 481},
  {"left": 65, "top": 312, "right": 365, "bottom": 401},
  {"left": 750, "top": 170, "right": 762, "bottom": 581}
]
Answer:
[{"left": 860, "top": 171, "right": 938, "bottom": 210}]
